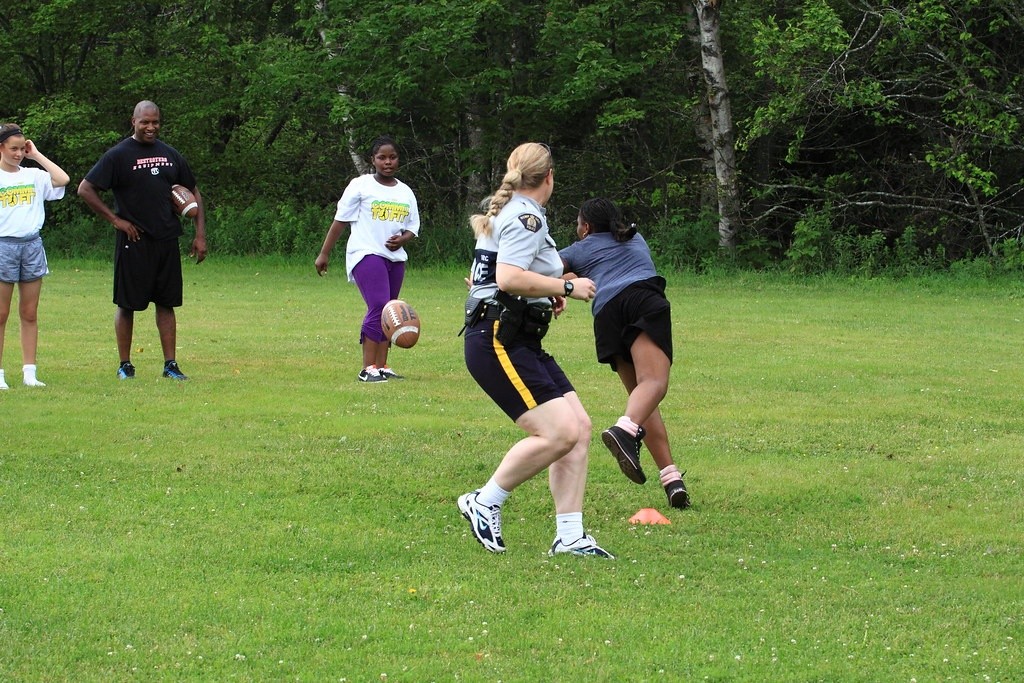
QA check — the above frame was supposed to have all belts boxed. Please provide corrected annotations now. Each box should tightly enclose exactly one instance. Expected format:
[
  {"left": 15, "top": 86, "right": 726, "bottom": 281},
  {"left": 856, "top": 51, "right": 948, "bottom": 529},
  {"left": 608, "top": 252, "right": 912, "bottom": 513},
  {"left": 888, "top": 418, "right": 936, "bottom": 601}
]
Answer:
[{"left": 478, "top": 306, "right": 504, "bottom": 319}]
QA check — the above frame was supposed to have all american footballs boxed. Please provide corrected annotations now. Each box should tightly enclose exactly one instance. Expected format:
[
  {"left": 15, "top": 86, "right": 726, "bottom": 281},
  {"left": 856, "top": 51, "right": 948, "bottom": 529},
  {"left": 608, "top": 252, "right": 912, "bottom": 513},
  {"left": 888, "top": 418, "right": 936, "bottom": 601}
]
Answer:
[
  {"left": 171, "top": 184, "right": 198, "bottom": 217},
  {"left": 380, "top": 300, "right": 420, "bottom": 349}
]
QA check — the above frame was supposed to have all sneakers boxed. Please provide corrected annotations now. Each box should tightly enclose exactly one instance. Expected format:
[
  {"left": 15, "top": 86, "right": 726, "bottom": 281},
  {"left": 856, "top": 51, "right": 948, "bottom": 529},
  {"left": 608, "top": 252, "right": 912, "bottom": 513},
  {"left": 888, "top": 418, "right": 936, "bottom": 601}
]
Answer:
[
  {"left": 458, "top": 488, "right": 506, "bottom": 555},
  {"left": 602, "top": 426, "right": 646, "bottom": 485},
  {"left": 358, "top": 364, "right": 388, "bottom": 383},
  {"left": 378, "top": 365, "right": 405, "bottom": 380},
  {"left": 117, "top": 363, "right": 135, "bottom": 380},
  {"left": 162, "top": 361, "right": 188, "bottom": 381},
  {"left": 547, "top": 531, "right": 614, "bottom": 561},
  {"left": 663, "top": 470, "right": 690, "bottom": 511}
]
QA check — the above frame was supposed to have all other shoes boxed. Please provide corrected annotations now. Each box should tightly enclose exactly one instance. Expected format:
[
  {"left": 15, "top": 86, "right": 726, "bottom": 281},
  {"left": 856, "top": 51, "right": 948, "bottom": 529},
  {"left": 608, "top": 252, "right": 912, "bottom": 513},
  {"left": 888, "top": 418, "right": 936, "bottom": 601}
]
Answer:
[
  {"left": 23, "top": 378, "right": 47, "bottom": 388},
  {"left": 0, "top": 381, "right": 9, "bottom": 390}
]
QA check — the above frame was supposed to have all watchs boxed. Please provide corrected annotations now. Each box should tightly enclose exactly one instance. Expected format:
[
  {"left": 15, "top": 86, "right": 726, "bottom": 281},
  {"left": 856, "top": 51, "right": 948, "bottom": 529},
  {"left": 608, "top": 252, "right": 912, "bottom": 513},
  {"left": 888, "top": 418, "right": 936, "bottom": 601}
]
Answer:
[{"left": 562, "top": 280, "right": 574, "bottom": 297}]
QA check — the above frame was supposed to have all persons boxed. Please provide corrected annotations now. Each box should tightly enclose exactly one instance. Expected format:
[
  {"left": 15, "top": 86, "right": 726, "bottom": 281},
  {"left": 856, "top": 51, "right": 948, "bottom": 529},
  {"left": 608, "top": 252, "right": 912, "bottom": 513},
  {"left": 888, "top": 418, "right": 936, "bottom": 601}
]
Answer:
[
  {"left": 462, "top": 198, "right": 690, "bottom": 510},
  {"left": 77, "top": 100, "right": 208, "bottom": 381},
  {"left": 455, "top": 141, "right": 616, "bottom": 563},
  {"left": 313, "top": 137, "right": 420, "bottom": 381},
  {"left": 0, "top": 122, "right": 71, "bottom": 391}
]
